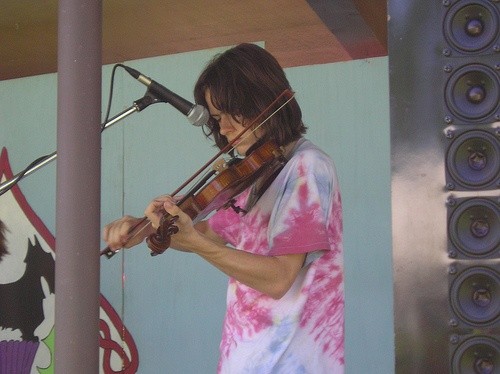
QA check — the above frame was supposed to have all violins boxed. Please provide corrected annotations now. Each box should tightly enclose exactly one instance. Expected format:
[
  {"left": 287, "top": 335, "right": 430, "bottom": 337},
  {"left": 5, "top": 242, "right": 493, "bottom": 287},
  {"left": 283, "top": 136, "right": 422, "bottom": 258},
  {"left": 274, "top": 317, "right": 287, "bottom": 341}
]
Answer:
[{"left": 142, "top": 120, "right": 301, "bottom": 256}]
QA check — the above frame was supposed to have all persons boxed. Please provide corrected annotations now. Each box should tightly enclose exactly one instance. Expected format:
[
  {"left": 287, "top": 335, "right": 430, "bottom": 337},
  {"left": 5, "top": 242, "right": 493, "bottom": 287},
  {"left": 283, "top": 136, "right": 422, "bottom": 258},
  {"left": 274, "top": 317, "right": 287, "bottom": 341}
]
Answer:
[{"left": 103, "top": 42, "right": 345, "bottom": 374}]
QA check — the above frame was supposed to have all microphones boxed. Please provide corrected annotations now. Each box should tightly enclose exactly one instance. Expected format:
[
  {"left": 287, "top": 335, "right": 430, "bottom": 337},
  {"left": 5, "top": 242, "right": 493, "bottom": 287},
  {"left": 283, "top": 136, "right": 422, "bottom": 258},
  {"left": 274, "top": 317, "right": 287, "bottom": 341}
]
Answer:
[{"left": 124, "top": 66, "right": 210, "bottom": 126}]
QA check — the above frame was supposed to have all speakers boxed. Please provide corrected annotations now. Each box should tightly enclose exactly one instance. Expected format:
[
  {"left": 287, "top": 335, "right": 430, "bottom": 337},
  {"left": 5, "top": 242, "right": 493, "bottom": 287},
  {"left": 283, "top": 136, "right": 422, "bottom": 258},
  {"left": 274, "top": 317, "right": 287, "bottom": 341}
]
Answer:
[{"left": 386, "top": 0, "right": 499, "bottom": 374}]
